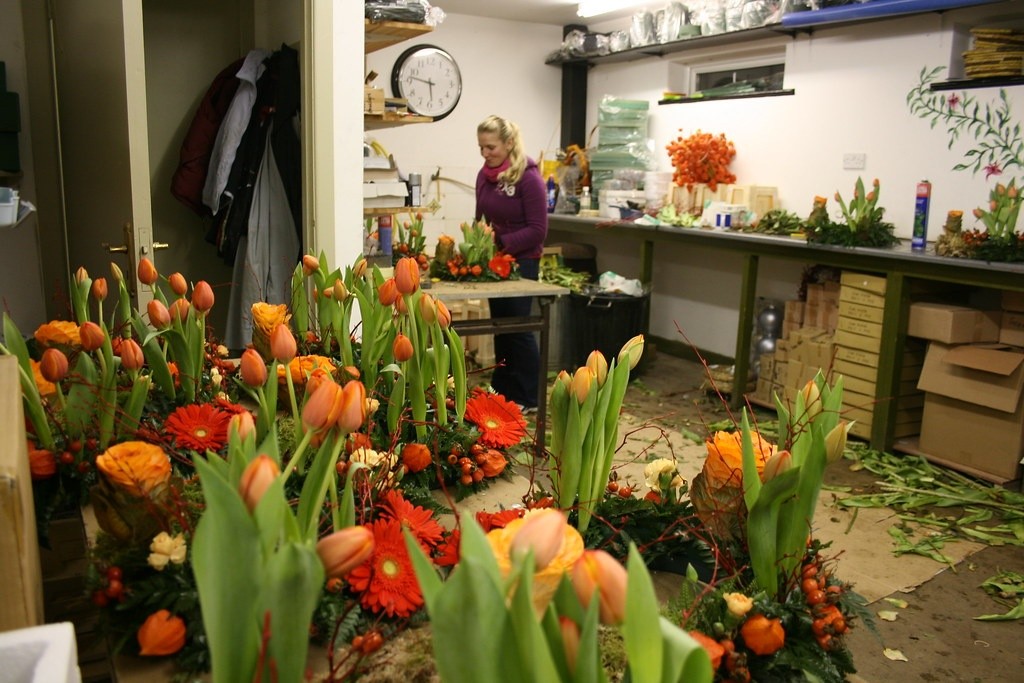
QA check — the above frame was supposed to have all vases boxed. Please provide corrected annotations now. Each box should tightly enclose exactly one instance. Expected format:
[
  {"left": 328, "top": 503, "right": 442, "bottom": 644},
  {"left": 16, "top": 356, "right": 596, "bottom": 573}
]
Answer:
[{"left": 685, "top": 183, "right": 708, "bottom": 218}]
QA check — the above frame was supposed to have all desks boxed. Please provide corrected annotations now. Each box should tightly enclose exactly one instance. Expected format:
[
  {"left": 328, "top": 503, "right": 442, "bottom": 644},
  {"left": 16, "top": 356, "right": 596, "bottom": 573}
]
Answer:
[
  {"left": 367, "top": 263, "right": 570, "bottom": 456},
  {"left": 546, "top": 211, "right": 1024, "bottom": 412}
]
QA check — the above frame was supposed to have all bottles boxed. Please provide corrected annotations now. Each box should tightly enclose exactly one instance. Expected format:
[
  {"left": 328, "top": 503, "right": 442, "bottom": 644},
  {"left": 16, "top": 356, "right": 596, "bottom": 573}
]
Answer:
[
  {"left": 377, "top": 214, "right": 394, "bottom": 256},
  {"left": 909, "top": 178, "right": 931, "bottom": 251},
  {"left": 541, "top": 174, "right": 560, "bottom": 213},
  {"left": 579, "top": 186, "right": 592, "bottom": 210}
]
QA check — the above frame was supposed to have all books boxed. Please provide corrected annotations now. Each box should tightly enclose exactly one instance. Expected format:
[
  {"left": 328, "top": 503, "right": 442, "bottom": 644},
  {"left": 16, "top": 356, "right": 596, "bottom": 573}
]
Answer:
[
  {"left": 363, "top": 168, "right": 400, "bottom": 181},
  {"left": 364, "top": 86, "right": 407, "bottom": 115},
  {"left": 664, "top": 81, "right": 756, "bottom": 99}
]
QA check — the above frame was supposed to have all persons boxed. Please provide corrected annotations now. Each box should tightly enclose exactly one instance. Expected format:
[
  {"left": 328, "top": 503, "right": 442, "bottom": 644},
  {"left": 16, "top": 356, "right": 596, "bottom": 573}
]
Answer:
[{"left": 460, "top": 115, "right": 547, "bottom": 414}]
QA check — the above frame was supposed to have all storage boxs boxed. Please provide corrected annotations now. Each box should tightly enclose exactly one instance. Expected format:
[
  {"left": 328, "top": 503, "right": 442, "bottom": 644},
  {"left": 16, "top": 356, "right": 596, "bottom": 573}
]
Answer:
[{"left": 754, "top": 272, "right": 1024, "bottom": 482}]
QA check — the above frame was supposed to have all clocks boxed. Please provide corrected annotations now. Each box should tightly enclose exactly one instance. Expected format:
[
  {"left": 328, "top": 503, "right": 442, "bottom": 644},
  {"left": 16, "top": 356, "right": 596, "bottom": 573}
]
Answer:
[{"left": 391, "top": 43, "right": 463, "bottom": 121}]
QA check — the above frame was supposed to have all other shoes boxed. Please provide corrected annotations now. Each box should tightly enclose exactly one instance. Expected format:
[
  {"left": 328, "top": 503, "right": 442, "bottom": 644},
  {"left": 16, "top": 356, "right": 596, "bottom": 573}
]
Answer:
[
  {"left": 518, "top": 404, "right": 538, "bottom": 416},
  {"left": 486, "top": 386, "right": 499, "bottom": 396}
]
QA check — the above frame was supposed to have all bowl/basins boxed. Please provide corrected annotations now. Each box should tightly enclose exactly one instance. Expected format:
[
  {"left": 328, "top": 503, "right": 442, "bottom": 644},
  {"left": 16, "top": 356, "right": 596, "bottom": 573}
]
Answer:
[{"left": 679, "top": 24, "right": 701, "bottom": 39}]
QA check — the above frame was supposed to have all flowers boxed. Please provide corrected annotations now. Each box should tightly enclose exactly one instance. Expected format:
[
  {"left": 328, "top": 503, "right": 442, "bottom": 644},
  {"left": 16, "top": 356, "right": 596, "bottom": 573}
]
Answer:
[{"left": 666, "top": 129, "right": 737, "bottom": 192}]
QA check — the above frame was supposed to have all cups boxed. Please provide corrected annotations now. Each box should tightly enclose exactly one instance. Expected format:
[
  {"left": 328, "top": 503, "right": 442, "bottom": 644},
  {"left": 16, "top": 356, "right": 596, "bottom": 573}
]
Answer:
[
  {"left": 714, "top": 213, "right": 731, "bottom": 231},
  {"left": 408, "top": 172, "right": 422, "bottom": 207}
]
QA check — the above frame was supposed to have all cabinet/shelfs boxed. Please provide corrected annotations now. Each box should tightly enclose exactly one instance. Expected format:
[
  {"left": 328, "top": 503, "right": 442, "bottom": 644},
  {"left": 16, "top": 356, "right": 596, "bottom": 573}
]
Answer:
[{"left": 364, "top": 18, "right": 434, "bottom": 133}]
